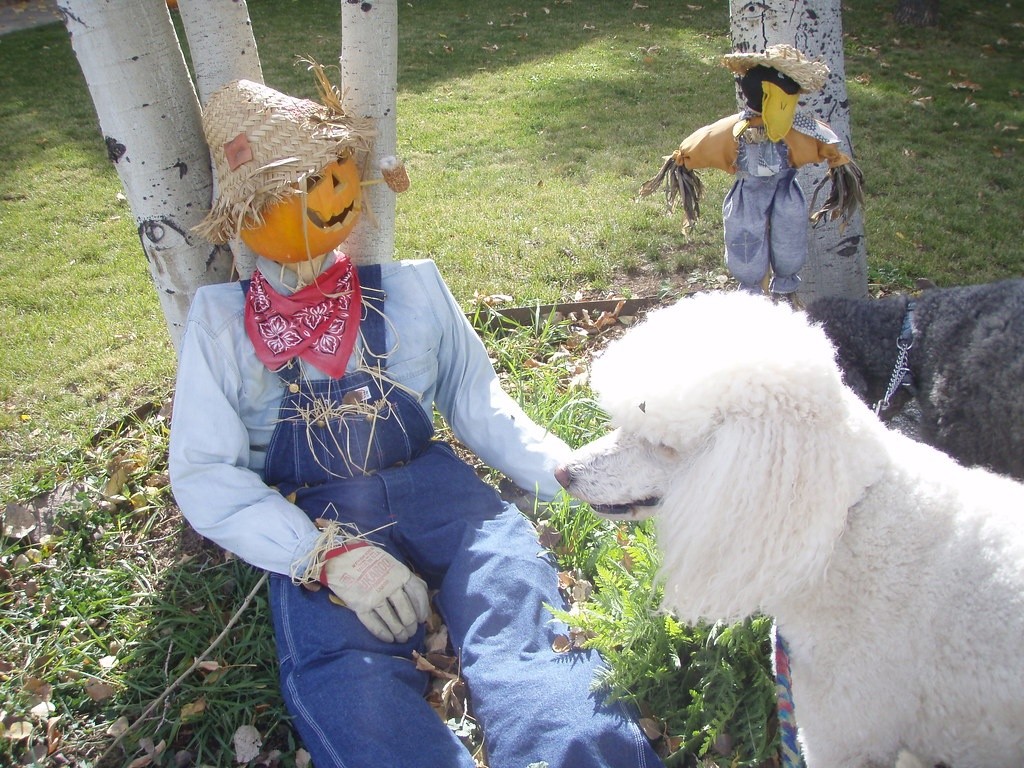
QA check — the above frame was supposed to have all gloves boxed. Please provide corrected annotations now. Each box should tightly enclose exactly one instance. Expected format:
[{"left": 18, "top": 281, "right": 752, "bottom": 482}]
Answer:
[{"left": 312, "top": 533, "right": 428, "bottom": 642}]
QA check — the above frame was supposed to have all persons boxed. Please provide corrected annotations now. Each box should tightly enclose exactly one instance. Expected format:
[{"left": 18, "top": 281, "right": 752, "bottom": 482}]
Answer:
[
  {"left": 638, "top": 42, "right": 866, "bottom": 311},
  {"left": 167, "top": 50, "right": 668, "bottom": 768}
]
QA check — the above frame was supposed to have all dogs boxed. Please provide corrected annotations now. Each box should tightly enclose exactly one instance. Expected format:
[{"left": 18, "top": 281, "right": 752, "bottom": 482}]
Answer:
[{"left": 553, "top": 277, "right": 1024, "bottom": 768}]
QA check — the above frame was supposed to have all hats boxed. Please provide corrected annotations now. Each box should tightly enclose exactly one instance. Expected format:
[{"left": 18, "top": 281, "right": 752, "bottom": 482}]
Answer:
[
  {"left": 188, "top": 55, "right": 380, "bottom": 245},
  {"left": 723, "top": 45, "right": 832, "bottom": 95}
]
